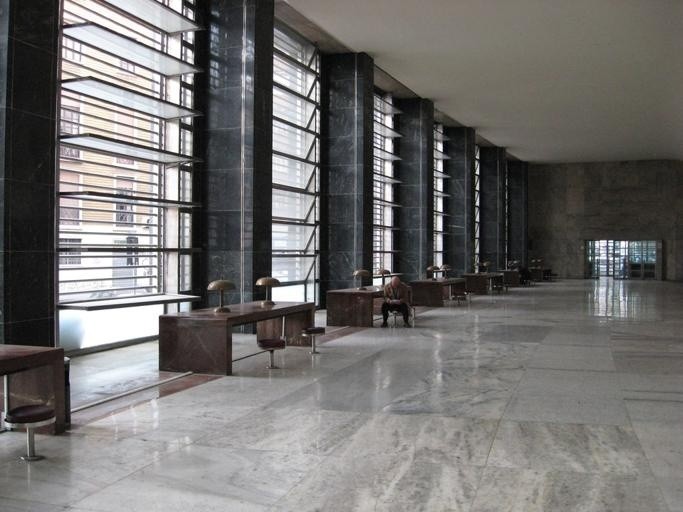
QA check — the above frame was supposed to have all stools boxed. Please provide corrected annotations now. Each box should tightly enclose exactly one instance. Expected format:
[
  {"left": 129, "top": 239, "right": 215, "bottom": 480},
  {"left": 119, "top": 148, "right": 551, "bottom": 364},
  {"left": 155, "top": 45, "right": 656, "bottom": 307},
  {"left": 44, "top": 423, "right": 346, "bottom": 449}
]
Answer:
[
  {"left": 387, "top": 310, "right": 402, "bottom": 328},
  {"left": 256, "top": 337, "right": 287, "bottom": 370},
  {"left": 408, "top": 267, "right": 559, "bottom": 307},
  {"left": 5, "top": 403, "right": 56, "bottom": 463},
  {"left": 298, "top": 325, "right": 327, "bottom": 356}
]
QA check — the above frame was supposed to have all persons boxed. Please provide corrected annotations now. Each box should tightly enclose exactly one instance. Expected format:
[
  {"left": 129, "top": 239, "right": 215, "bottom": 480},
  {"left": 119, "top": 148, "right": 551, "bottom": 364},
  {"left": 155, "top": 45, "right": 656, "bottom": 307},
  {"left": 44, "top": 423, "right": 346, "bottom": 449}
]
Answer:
[{"left": 380, "top": 275, "right": 412, "bottom": 328}]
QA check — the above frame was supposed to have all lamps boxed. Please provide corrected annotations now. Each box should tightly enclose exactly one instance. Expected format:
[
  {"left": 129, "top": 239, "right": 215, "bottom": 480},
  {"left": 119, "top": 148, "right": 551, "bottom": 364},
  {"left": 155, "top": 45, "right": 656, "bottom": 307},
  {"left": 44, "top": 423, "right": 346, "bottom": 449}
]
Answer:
[
  {"left": 352, "top": 268, "right": 370, "bottom": 291},
  {"left": 375, "top": 269, "right": 392, "bottom": 288},
  {"left": 254, "top": 274, "right": 281, "bottom": 306},
  {"left": 207, "top": 274, "right": 231, "bottom": 313}
]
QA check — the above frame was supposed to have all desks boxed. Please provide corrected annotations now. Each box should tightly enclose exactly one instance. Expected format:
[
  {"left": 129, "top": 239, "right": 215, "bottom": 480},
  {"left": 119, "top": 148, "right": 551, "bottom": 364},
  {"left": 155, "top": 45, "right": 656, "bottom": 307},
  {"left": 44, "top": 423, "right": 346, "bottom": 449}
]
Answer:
[
  {"left": 159, "top": 298, "right": 318, "bottom": 376},
  {"left": 325, "top": 285, "right": 414, "bottom": 328},
  {"left": 0, "top": 343, "right": 68, "bottom": 444}
]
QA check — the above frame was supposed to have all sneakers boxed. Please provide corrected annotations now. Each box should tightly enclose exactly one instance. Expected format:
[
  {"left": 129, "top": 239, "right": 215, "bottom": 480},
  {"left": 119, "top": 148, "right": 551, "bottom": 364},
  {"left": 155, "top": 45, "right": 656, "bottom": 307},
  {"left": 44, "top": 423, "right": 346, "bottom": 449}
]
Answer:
[
  {"left": 383, "top": 321, "right": 387, "bottom": 327},
  {"left": 404, "top": 323, "right": 411, "bottom": 328}
]
